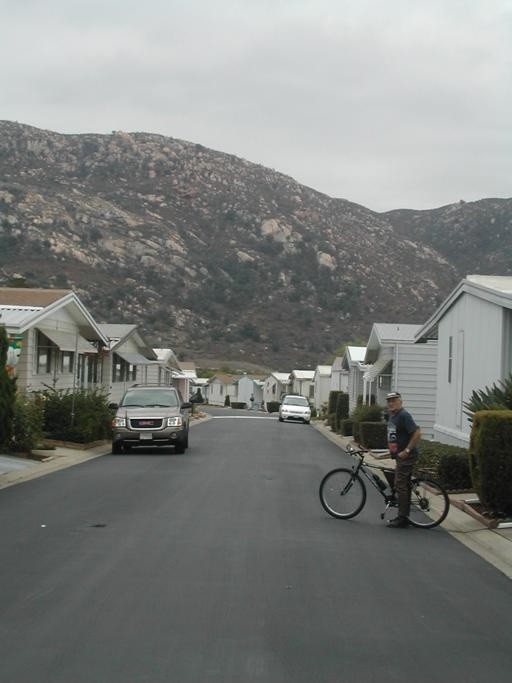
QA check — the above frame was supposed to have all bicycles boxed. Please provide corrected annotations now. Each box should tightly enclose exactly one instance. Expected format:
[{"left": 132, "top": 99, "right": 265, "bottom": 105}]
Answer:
[{"left": 317, "top": 444, "right": 451, "bottom": 528}]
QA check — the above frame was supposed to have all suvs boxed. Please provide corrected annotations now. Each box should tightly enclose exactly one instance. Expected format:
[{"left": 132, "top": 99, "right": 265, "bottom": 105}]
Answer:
[
  {"left": 275, "top": 393, "right": 312, "bottom": 423},
  {"left": 108, "top": 381, "right": 193, "bottom": 455}
]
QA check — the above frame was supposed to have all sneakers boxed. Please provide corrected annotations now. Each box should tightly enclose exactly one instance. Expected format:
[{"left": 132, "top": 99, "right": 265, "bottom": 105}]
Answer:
[{"left": 385, "top": 516, "right": 408, "bottom": 528}]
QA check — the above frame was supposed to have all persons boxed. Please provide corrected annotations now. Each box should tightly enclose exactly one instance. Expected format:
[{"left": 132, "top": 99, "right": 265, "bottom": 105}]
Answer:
[{"left": 383, "top": 389, "right": 421, "bottom": 528}]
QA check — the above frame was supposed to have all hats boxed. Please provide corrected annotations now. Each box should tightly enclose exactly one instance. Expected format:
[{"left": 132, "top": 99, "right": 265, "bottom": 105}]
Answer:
[{"left": 386, "top": 391, "right": 401, "bottom": 400}]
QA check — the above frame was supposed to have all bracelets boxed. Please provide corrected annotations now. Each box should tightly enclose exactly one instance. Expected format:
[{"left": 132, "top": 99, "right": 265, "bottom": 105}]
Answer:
[{"left": 404, "top": 447, "right": 410, "bottom": 453}]
